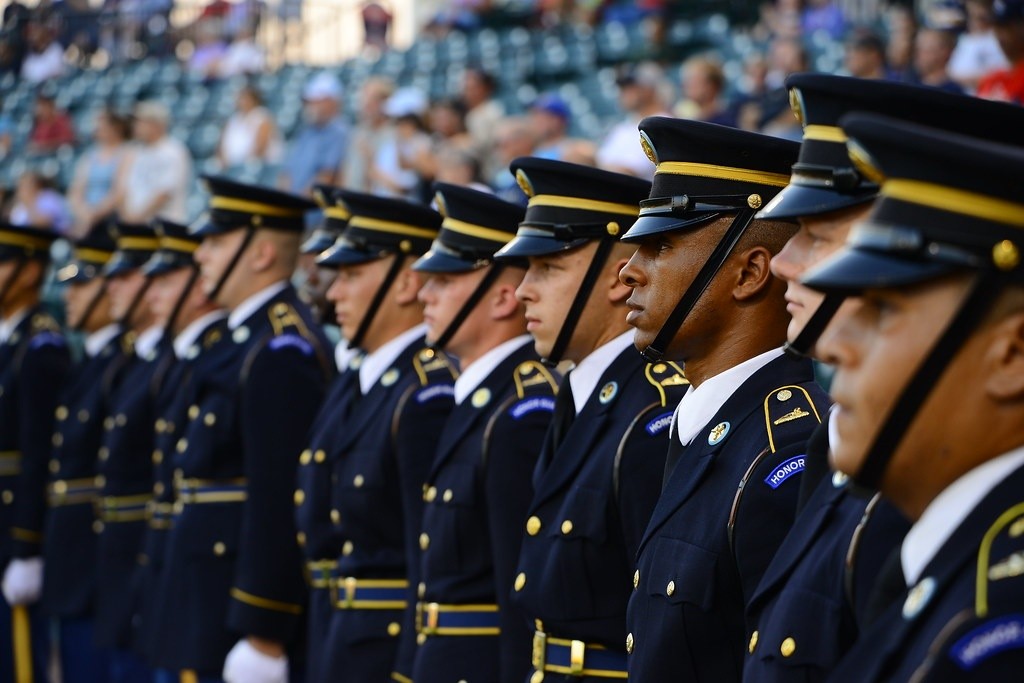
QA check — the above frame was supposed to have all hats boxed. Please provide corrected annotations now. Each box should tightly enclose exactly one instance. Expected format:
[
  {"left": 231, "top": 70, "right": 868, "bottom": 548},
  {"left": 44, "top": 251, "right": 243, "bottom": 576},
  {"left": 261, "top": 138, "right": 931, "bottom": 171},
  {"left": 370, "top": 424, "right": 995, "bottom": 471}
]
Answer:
[
  {"left": 620, "top": 117, "right": 801, "bottom": 241},
  {"left": 185, "top": 173, "right": 305, "bottom": 239},
  {"left": 493, "top": 157, "right": 651, "bottom": 257},
  {"left": 103, "top": 225, "right": 151, "bottom": 276},
  {"left": 300, "top": 188, "right": 350, "bottom": 252},
  {"left": 754, "top": 71, "right": 1024, "bottom": 222},
  {"left": 137, "top": 217, "right": 202, "bottom": 275},
  {"left": 314, "top": 190, "right": 444, "bottom": 268},
  {"left": 379, "top": 88, "right": 428, "bottom": 119},
  {"left": 300, "top": 72, "right": 342, "bottom": 103},
  {"left": 800, "top": 113, "right": 1024, "bottom": 293},
  {"left": 127, "top": 102, "right": 168, "bottom": 123},
  {"left": 408, "top": 182, "right": 529, "bottom": 273},
  {"left": 62, "top": 219, "right": 126, "bottom": 287},
  {"left": 1, "top": 224, "right": 59, "bottom": 264}
]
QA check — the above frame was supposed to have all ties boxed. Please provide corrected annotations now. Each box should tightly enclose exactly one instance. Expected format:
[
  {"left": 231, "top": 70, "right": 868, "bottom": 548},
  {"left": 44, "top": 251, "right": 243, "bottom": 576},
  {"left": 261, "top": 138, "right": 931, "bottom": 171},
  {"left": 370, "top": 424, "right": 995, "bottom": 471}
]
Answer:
[
  {"left": 794, "top": 412, "right": 831, "bottom": 518},
  {"left": 543, "top": 371, "right": 577, "bottom": 477},
  {"left": 661, "top": 413, "right": 691, "bottom": 493},
  {"left": 858, "top": 551, "right": 908, "bottom": 633}
]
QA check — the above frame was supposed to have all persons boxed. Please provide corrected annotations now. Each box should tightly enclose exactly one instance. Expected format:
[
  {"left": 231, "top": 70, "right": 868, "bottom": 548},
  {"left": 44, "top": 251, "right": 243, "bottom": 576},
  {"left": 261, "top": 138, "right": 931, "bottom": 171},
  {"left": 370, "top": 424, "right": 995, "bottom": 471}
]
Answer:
[{"left": 0, "top": 0, "right": 1024, "bottom": 683}]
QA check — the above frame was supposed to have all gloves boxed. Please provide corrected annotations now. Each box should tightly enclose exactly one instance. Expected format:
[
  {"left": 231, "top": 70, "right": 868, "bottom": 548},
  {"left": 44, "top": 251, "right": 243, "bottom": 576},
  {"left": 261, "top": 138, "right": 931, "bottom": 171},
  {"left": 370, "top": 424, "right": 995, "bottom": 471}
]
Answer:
[
  {"left": 221, "top": 640, "right": 288, "bottom": 682},
  {"left": 1, "top": 558, "right": 44, "bottom": 606}
]
